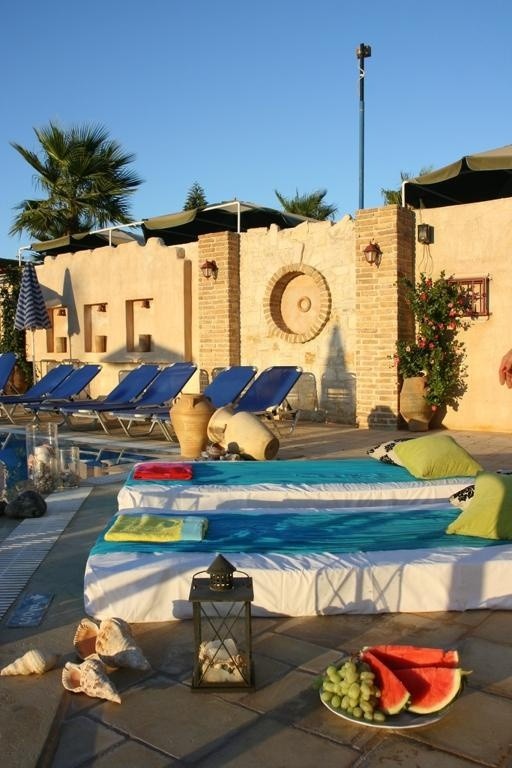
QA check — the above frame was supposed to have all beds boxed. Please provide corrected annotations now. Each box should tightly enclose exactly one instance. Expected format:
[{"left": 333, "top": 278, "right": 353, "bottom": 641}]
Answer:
[
  {"left": 84, "top": 504, "right": 512, "bottom": 624},
  {"left": 117, "top": 457, "right": 476, "bottom": 511}
]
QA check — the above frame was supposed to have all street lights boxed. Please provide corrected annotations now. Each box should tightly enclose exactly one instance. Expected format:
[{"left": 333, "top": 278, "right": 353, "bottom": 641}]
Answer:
[{"left": 357, "top": 43, "right": 373, "bottom": 208}]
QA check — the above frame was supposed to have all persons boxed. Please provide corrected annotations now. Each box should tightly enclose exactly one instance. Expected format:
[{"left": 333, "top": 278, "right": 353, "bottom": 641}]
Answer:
[{"left": 499, "top": 349, "right": 512, "bottom": 390}]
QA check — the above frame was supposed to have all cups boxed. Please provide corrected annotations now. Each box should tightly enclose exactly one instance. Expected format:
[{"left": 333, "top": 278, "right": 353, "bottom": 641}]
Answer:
[{"left": 26, "top": 422, "right": 79, "bottom": 495}]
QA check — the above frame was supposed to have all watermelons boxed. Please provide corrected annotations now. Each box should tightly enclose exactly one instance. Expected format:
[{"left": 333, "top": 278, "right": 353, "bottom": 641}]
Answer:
[{"left": 358, "top": 644, "right": 474, "bottom": 717}]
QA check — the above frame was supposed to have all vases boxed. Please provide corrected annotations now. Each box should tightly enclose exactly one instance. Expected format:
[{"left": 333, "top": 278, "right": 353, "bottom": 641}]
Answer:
[{"left": 399, "top": 377, "right": 437, "bottom": 430}]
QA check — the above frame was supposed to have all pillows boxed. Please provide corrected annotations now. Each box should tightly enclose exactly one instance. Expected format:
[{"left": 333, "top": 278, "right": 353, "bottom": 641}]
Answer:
[
  {"left": 448, "top": 469, "right": 512, "bottom": 511},
  {"left": 366, "top": 438, "right": 414, "bottom": 465},
  {"left": 446, "top": 470, "right": 512, "bottom": 539},
  {"left": 393, "top": 436, "right": 483, "bottom": 479}
]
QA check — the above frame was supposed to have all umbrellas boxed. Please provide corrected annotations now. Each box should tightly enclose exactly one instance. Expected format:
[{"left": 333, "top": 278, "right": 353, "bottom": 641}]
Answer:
[{"left": 12, "top": 252, "right": 51, "bottom": 387}]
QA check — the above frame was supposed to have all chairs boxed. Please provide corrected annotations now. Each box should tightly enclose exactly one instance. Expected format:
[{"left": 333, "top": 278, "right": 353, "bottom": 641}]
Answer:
[
  {"left": 2, "top": 364, "right": 76, "bottom": 418},
  {"left": 146, "top": 366, "right": 302, "bottom": 444},
  {"left": 1, "top": 364, "right": 103, "bottom": 424},
  {"left": 109, "top": 365, "right": 258, "bottom": 440},
  {"left": 60, "top": 361, "right": 199, "bottom": 439},
  {"left": 1, "top": 353, "right": 19, "bottom": 390},
  {"left": 24, "top": 363, "right": 162, "bottom": 430}
]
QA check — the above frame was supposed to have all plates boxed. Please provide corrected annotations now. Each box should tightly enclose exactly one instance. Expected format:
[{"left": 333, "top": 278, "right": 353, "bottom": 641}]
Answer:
[{"left": 318, "top": 687, "right": 453, "bottom": 730}]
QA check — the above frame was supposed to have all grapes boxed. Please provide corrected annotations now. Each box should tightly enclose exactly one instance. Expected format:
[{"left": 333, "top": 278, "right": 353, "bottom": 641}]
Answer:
[{"left": 308, "top": 662, "right": 386, "bottom": 721}]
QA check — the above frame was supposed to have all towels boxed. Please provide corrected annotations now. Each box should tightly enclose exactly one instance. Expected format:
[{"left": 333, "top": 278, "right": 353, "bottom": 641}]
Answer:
[
  {"left": 105, "top": 515, "right": 208, "bottom": 542},
  {"left": 134, "top": 463, "right": 193, "bottom": 480}
]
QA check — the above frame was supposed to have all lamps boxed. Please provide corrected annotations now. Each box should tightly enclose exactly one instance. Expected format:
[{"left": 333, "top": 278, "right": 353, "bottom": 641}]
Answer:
[
  {"left": 200, "top": 259, "right": 217, "bottom": 280},
  {"left": 363, "top": 240, "right": 381, "bottom": 265}
]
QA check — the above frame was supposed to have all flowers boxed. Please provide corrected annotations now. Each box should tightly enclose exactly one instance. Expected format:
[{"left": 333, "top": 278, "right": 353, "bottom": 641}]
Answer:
[{"left": 386, "top": 270, "right": 472, "bottom": 411}]
